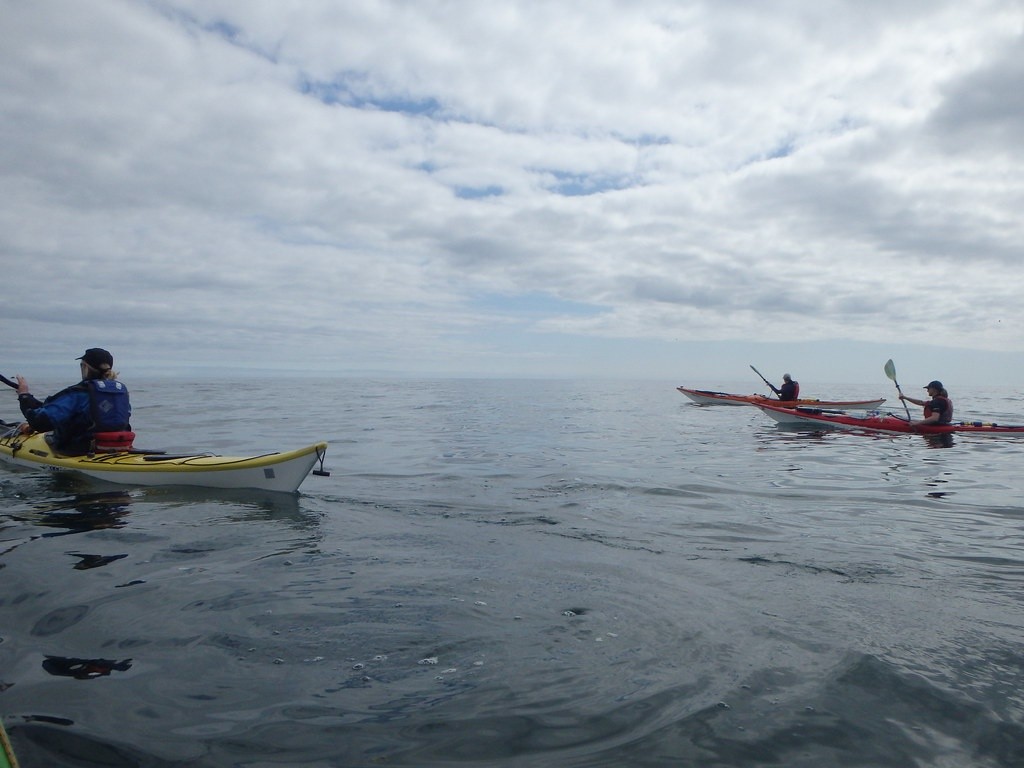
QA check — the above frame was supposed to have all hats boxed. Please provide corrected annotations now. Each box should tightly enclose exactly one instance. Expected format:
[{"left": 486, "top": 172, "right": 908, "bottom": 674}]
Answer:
[
  {"left": 923, "top": 381, "right": 943, "bottom": 389},
  {"left": 75, "top": 347, "right": 113, "bottom": 375},
  {"left": 783, "top": 373, "right": 792, "bottom": 380}
]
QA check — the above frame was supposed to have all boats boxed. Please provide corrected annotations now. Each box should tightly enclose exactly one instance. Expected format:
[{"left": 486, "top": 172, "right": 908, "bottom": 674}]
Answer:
[
  {"left": 0, "top": 415, "right": 332, "bottom": 494},
  {"left": 676, "top": 384, "right": 887, "bottom": 411},
  {"left": 749, "top": 397, "right": 1023, "bottom": 437}
]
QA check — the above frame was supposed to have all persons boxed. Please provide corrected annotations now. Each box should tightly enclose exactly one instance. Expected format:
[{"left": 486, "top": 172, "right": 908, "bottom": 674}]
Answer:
[
  {"left": 899, "top": 381, "right": 953, "bottom": 426},
  {"left": 766, "top": 373, "right": 799, "bottom": 400},
  {"left": 16, "top": 348, "right": 131, "bottom": 457}
]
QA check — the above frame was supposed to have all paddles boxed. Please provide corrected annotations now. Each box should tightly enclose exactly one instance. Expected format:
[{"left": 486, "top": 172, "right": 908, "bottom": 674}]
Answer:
[
  {"left": 0, "top": 374, "right": 46, "bottom": 405},
  {"left": 750, "top": 364, "right": 779, "bottom": 397},
  {"left": 884, "top": 359, "right": 910, "bottom": 420},
  {"left": 782, "top": 405, "right": 868, "bottom": 420},
  {"left": 696, "top": 390, "right": 746, "bottom": 398}
]
